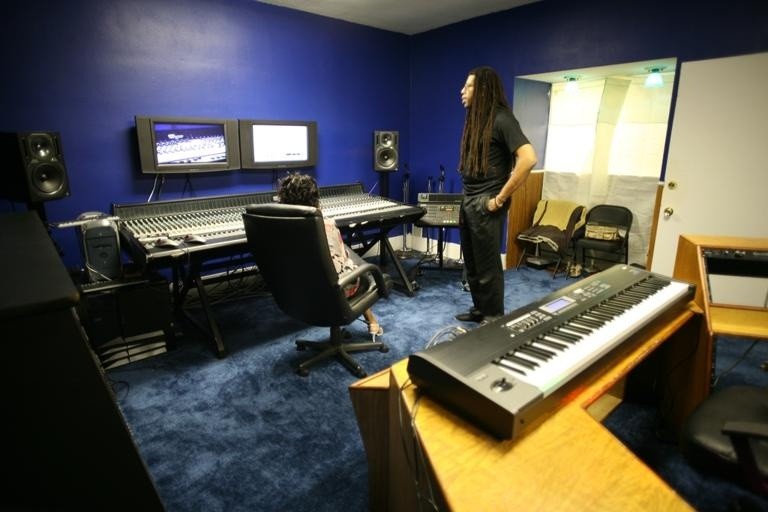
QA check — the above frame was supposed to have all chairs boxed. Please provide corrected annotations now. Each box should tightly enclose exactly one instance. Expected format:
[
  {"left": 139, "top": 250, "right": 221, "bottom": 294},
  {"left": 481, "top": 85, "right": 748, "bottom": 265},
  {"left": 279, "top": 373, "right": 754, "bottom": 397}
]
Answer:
[
  {"left": 242, "top": 200, "right": 388, "bottom": 376},
  {"left": 567, "top": 203, "right": 634, "bottom": 274},
  {"left": 516, "top": 199, "right": 580, "bottom": 280}
]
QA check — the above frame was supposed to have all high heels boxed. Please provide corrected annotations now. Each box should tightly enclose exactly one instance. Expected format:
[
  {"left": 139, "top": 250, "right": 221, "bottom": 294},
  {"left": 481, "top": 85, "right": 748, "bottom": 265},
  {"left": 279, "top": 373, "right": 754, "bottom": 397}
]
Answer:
[{"left": 366, "top": 322, "right": 384, "bottom": 344}]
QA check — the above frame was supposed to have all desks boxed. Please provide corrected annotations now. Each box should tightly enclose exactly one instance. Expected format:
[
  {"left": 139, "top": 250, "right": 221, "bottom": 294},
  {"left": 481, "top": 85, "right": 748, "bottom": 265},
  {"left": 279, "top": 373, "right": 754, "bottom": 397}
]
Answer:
[
  {"left": 672, "top": 232, "right": 767, "bottom": 409},
  {"left": 350, "top": 284, "right": 702, "bottom": 512}
]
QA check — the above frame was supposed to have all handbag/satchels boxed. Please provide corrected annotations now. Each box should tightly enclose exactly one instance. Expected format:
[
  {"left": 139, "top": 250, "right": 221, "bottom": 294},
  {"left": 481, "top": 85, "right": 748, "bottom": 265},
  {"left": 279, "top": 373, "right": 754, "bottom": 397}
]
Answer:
[{"left": 584, "top": 224, "right": 618, "bottom": 242}]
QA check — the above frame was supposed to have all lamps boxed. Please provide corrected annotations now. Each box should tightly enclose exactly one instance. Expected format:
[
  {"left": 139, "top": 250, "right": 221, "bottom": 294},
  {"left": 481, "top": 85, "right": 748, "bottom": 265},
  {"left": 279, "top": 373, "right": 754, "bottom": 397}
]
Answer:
[{"left": 646, "top": 68, "right": 663, "bottom": 91}]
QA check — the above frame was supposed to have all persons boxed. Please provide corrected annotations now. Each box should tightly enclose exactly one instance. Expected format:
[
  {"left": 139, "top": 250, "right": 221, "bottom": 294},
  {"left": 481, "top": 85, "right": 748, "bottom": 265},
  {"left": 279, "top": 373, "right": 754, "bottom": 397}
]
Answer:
[
  {"left": 456, "top": 66, "right": 538, "bottom": 326},
  {"left": 271, "top": 171, "right": 384, "bottom": 344}
]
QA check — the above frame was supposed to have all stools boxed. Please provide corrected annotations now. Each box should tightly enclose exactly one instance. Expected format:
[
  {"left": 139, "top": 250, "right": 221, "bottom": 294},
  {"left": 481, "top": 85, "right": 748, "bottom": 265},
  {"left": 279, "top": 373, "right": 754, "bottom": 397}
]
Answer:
[{"left": 681, "top": 379, "right": 766, "bottom": 510}]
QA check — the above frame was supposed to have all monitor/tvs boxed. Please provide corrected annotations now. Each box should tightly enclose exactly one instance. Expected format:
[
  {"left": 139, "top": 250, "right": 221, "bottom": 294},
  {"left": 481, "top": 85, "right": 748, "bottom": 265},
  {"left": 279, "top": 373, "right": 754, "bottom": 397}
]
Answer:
[
  {"left": 135, "top": 115, "right": 241, "bottom": 174},
  {"left": 239, "top": 119, "right": 318, "bottom": 170}
]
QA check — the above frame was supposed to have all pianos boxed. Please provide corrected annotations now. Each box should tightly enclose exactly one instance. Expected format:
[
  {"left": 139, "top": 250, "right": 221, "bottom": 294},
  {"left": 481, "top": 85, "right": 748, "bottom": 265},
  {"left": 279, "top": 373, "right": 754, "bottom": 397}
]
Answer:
[{"left": 408, "top": 262, "right": 696, "bottom": 443}]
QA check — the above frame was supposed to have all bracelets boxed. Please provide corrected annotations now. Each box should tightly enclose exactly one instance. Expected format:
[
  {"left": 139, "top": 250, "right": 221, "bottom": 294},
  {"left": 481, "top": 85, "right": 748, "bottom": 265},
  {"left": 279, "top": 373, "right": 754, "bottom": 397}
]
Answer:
[
  {"left": 497, "top": 195, "right": 507, "bottom": 202},
  {"left": 495, "top": 197, "right": 504, "bottom": 208}
]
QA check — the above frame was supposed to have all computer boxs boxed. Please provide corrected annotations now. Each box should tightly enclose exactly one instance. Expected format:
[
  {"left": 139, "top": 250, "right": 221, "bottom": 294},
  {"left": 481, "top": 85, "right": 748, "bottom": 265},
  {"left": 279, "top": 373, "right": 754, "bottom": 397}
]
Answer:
[{"left": 80, "top": 211, "right": 121, "bottom": 281}]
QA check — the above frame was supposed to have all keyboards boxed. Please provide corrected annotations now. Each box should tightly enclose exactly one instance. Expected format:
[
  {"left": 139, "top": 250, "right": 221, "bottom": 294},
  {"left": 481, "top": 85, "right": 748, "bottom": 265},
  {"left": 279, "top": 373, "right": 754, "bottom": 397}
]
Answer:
[{"left": 80, "top": 276, "right": 151, "bottom": 294}]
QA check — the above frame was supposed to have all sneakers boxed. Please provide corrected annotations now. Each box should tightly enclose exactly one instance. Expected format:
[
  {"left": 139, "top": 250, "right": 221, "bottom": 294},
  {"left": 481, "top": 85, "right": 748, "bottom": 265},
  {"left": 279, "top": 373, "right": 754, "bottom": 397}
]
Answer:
[
  {"left": 477, "top": 315, "right": 502, "bottom": 329},
  {"left": 455, "top": 308, "right": 483, "bottom": 324}
]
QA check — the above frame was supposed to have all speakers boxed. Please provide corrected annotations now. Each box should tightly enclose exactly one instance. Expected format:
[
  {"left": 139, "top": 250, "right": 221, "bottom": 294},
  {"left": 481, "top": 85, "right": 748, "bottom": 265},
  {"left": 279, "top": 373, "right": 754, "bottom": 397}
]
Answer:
[
  {"left": 373, "top": 130, "right": 400, "bottom": 172},
  {"left": 0, "top": 130, "right": 72, "bottom": 204}
]
